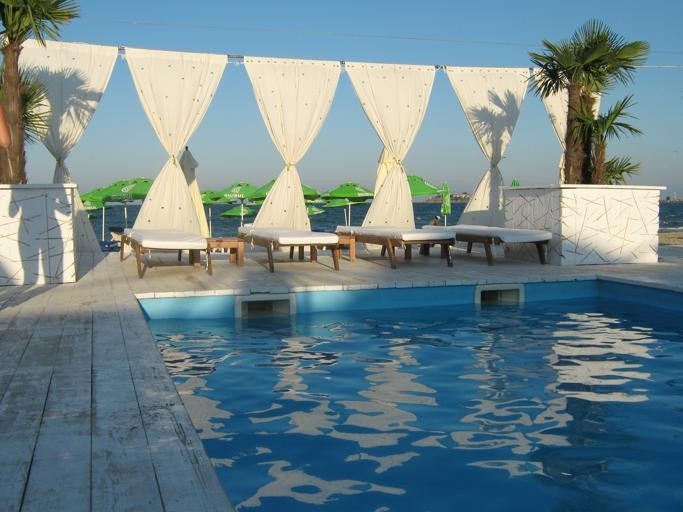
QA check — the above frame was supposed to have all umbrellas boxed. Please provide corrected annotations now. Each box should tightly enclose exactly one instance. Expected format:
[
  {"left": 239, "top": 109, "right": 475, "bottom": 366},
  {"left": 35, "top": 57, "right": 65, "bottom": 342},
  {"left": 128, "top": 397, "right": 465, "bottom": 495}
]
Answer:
[{"left": 440, "top": 181, "right": 451, "bottom": 225}]
